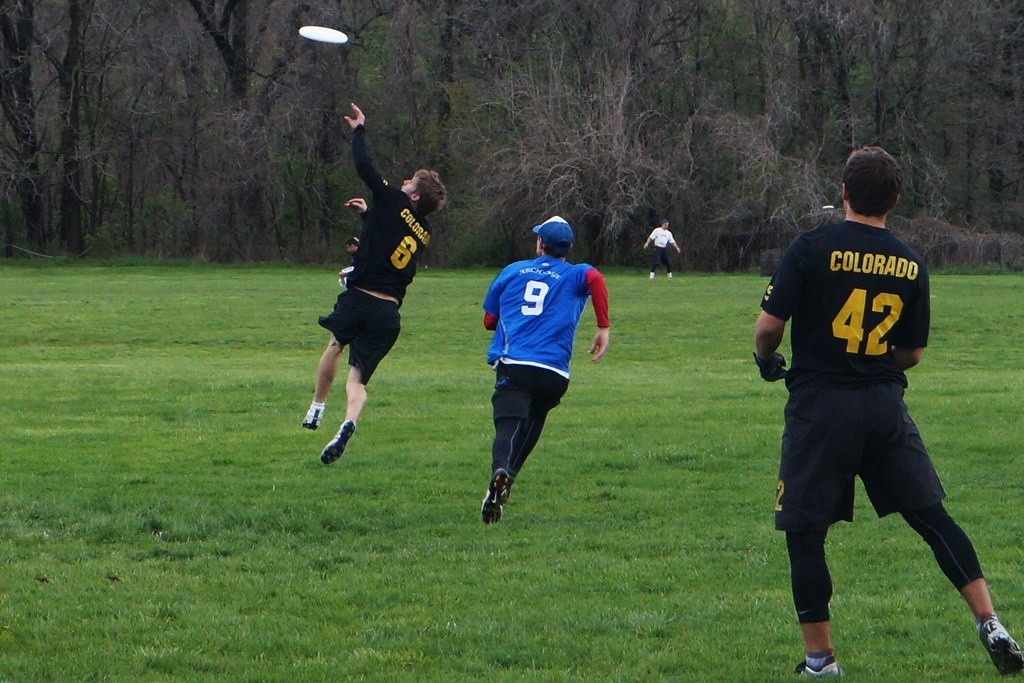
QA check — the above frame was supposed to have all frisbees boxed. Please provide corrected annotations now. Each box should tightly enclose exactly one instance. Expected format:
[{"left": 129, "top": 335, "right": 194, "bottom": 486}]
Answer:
[{"left": 300, "top": 23, "right": 348, "bottom": 44}]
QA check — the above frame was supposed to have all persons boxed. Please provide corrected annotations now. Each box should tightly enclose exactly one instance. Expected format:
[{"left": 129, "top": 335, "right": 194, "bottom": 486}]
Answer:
[
  {"left": 643, "top": 218, "right": 681, "bottom": 279},
  {"left": 300, "top": 103, "right": 448, "bottom": 466},
  {"left": 750, "top": 145, "right": 1024, "bottom": 677},
  {"left": 481, "top": 215, "right": 611, "bottom": 524}
]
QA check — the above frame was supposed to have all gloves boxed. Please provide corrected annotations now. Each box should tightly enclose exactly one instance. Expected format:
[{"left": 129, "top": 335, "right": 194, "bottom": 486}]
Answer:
[{"left": 753, "top": 351, "right": 787, "bottom": 382}]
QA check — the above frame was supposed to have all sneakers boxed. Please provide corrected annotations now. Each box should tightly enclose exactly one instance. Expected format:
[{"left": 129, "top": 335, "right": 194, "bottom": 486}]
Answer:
[
  {"left": 794, "top": 661, "right": 846, "bottom": 680},
  {"left": 320, "top": 419, "right": 356, "bottom": 464},
  {"left": 481, "top": 468, "right": 509, "bottom": 525},
  {"left": 977, "top": 612, "right": 1024, "bottom": 677},
  {"left": 302, "top": 409, "right": 323, "bottom": 431}
]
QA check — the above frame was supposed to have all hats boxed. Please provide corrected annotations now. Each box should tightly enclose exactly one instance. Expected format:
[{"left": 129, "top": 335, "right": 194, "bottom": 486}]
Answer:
[{"left": 533, "top": 216, "right": 574, "bottom": 249}]
